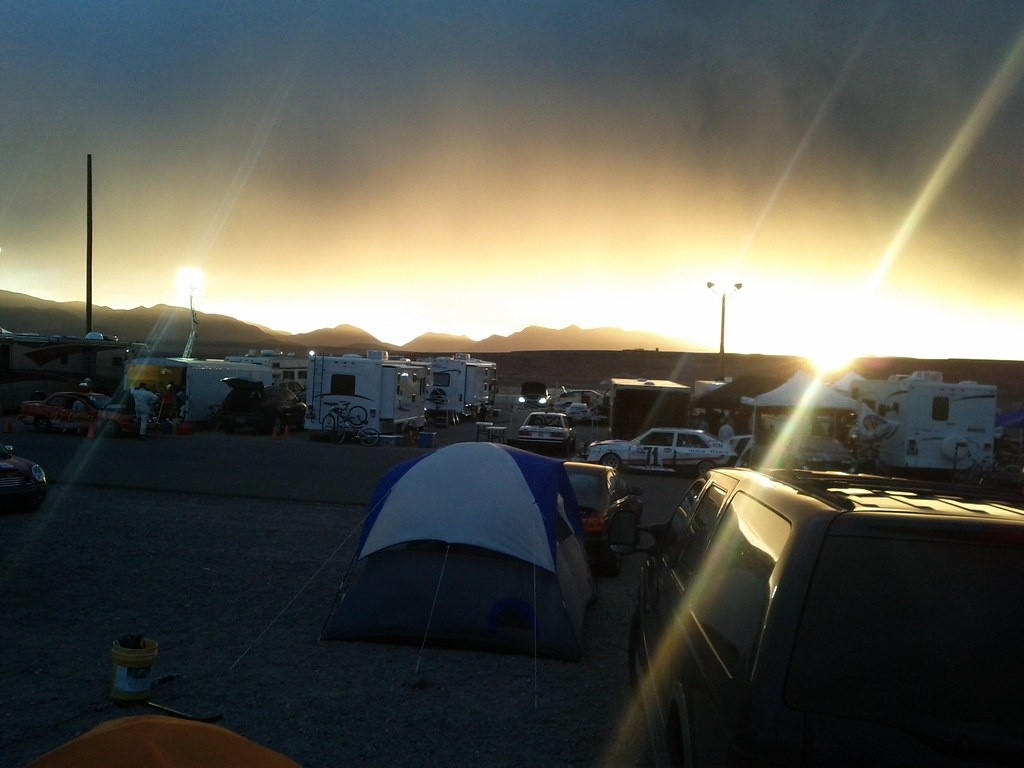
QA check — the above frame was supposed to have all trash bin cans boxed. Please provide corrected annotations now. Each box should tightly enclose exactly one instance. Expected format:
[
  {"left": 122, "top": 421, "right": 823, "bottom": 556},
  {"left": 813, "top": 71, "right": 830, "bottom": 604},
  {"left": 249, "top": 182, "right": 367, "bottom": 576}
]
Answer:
[{"left": 420, "top": 431, "right": 436, "bottom": 447}]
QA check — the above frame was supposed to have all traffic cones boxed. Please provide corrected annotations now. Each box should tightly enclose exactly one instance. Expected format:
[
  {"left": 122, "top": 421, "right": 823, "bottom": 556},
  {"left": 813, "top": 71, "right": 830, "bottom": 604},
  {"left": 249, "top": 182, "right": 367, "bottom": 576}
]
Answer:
[
  {"left": 271, "top": 425, "right": 279, "bottom": 441},
  {"left": 283, "top": 424, "right": 289, "bottom": 439},
  {"left": 2, "top": 420, "right": 11, "bottom": 434},
  {"left": 85, "top": 422, "right": 94, "bottom": 439}
]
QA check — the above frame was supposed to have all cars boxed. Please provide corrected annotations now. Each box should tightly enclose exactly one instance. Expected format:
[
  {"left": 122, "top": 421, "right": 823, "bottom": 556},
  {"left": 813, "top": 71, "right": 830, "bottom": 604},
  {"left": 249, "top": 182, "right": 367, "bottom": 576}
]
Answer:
[
  {"left": 516, "top": 411, "right": 576, "bottom": 455},
  {"left": 584, "top": 427, "right": 738, "bottom": 480},
  {"left": 561, "top": 461, "right": 644, "bottom": 576},
  {"left": 0, "top": 441, "right": 48, "bottom": 506},
  {"left": 18, "top": 389, "right": 160, "bottom": 440},
  {"left": 550, "top": 389, "right": 602, "bottom": 408},
  {"left": 564, "top": 403, "right": 592, "bottom": 423},
  {"left": 784, "top": 433, "right": 857, "bottom": 475}
]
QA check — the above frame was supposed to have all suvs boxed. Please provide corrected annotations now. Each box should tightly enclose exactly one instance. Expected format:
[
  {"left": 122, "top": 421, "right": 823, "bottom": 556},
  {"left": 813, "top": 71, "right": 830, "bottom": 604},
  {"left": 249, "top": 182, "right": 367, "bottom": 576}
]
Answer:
[{"left": 607, "top": 467, "right": 1024, "bottom": 768}]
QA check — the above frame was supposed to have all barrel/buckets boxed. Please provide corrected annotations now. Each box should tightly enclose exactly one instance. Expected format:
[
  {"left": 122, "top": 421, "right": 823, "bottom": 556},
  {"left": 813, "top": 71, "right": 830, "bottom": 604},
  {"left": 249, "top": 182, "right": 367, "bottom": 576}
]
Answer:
[
  {"left": 109, "top": 638, "right": 159, "bottom": 702},
  {"left": 418, "top": 432, "right": 435, "bottom": 448}
]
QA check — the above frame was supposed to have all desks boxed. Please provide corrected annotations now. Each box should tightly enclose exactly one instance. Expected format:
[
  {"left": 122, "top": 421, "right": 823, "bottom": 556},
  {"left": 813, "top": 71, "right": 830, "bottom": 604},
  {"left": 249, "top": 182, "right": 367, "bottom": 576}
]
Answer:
[{"left": 475, "top": 420, "right": 507, "bottom": 444}]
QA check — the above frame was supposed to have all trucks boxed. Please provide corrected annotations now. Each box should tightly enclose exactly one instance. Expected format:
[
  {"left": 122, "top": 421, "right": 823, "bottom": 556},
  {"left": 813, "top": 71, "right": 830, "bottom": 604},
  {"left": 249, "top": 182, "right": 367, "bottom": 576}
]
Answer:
[{"left": 517, "top": 380, "right": 551, "bottom": 409}]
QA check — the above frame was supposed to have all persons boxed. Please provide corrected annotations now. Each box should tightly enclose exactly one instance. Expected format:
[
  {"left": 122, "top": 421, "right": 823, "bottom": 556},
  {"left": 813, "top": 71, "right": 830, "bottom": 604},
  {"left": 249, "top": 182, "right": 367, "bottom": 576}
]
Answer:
[
  {"left": 130, "top": 382, "right": 158, "bottom": 440},
  {"left": 269, "top": 383, "right": 298, "bottom": 440},
  {"left": 697, "top": 409, "right": 747, "bottom": 440}
]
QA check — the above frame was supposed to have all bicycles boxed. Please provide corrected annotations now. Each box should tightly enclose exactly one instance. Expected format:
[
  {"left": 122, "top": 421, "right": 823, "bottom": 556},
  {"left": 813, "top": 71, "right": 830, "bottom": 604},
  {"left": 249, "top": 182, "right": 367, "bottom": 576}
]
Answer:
[{"left": 319, "top": 400, "right": 381, "bottom": 447}]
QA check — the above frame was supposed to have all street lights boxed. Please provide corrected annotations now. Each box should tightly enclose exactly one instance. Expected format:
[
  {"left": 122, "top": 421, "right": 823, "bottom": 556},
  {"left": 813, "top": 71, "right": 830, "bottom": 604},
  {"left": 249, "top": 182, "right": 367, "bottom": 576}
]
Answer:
[{"left": 706, "top": 280, "right": 743, "bottom": 417}]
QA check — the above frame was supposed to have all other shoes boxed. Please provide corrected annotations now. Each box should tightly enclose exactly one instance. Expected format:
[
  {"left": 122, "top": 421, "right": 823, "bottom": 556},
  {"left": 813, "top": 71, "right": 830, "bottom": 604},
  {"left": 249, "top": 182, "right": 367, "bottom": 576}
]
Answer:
[{"left": 140, "top": 435, "right": 145, "bottom": 440}]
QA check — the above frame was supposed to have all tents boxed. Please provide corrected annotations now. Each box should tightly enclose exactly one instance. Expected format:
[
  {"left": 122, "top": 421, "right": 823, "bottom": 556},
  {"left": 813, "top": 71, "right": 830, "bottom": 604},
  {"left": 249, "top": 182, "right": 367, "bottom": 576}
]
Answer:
[
  {"left": 690, "top": 361, "right": 814, "bottom": 436},
  {"left": 826, "top": 371, "right": 868, "bottom": 394},
  {"left": 741, "top": 368, "right": 860, "bottom": 473},
  {"left": 314, "top": 442, "right": 601, "bottom": 663}
]
